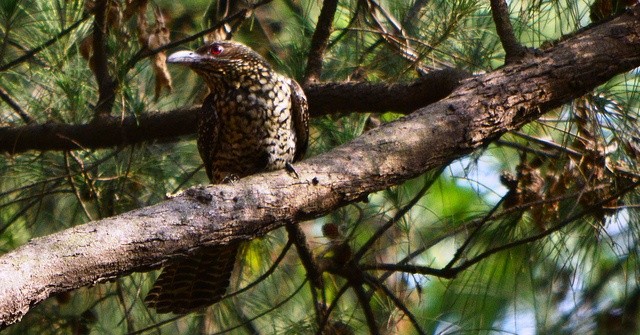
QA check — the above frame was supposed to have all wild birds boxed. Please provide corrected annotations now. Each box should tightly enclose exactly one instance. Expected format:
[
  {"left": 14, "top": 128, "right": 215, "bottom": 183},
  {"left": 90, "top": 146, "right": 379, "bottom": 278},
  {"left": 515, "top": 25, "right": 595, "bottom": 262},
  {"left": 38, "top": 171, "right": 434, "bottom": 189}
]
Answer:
[{"left": 143, "top": 39, "right": 310, "bottom": 314}]
